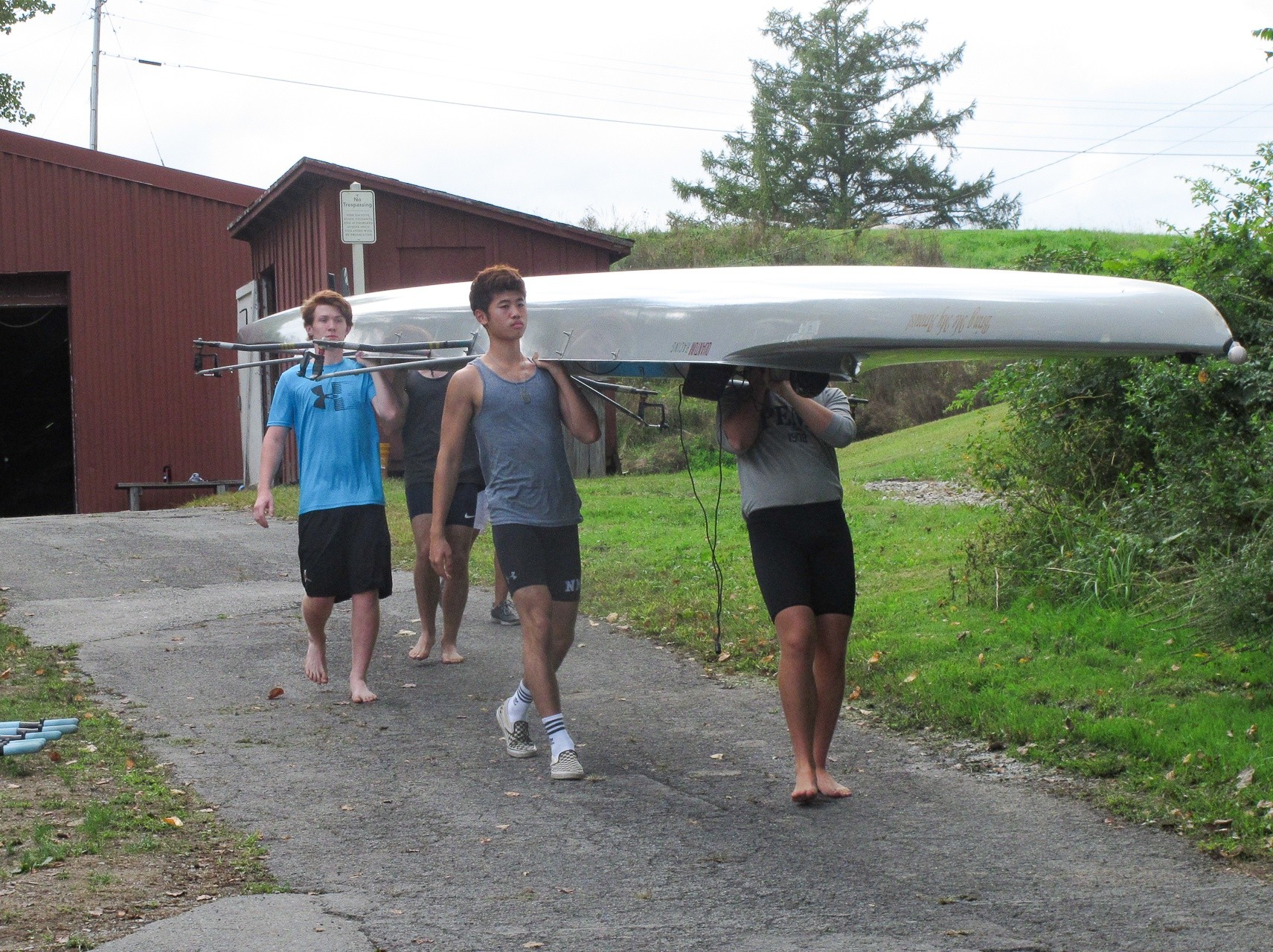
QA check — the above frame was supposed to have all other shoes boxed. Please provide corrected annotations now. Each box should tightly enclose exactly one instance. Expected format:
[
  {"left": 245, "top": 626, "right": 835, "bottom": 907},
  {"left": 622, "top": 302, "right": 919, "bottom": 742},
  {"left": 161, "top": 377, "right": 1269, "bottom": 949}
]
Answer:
[
  {"left": 550, "top": 750, "right": 584, "bottom": 778},
  {"left": 495, "top": 697, "right": 537, "bottom": 758}
]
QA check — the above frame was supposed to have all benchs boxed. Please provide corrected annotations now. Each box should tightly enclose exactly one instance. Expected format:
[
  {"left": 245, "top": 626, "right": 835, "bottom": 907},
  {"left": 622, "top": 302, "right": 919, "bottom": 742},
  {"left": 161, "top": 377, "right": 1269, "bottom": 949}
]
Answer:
[{"left": 115, "top": 479, "right": 243, "bottom": 512}]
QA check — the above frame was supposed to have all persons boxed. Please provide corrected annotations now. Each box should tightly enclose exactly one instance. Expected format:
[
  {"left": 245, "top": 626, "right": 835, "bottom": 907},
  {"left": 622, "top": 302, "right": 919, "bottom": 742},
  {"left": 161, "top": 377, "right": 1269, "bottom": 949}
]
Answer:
[
  {"left": 251, "top": 289, "right": 394, "bottom": 702},
  {"left": 439, "top": 483, "right": 521, "bottom": 625},
  {"left": 391, "top": 363, "right": 481, "bottom": 663},
  {"left": 715, "top": 376, "right": 858, "bottom": 800},
  {"left": 430, "top": 264, "right": 605, "bottom": 780}
]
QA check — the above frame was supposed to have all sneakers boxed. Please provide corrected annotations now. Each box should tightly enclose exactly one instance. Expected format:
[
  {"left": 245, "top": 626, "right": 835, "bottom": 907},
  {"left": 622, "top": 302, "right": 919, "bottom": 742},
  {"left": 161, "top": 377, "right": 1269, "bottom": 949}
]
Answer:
[{"left": 491, "top": 600, "right": 520, "bottom": 625}]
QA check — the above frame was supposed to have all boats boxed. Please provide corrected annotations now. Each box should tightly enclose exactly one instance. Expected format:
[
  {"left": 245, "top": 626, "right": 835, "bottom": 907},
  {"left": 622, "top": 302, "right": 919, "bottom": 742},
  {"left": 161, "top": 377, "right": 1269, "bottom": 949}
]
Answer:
[{"left": 234, "top": 264, "right": 1251, "bottom": 384}]
